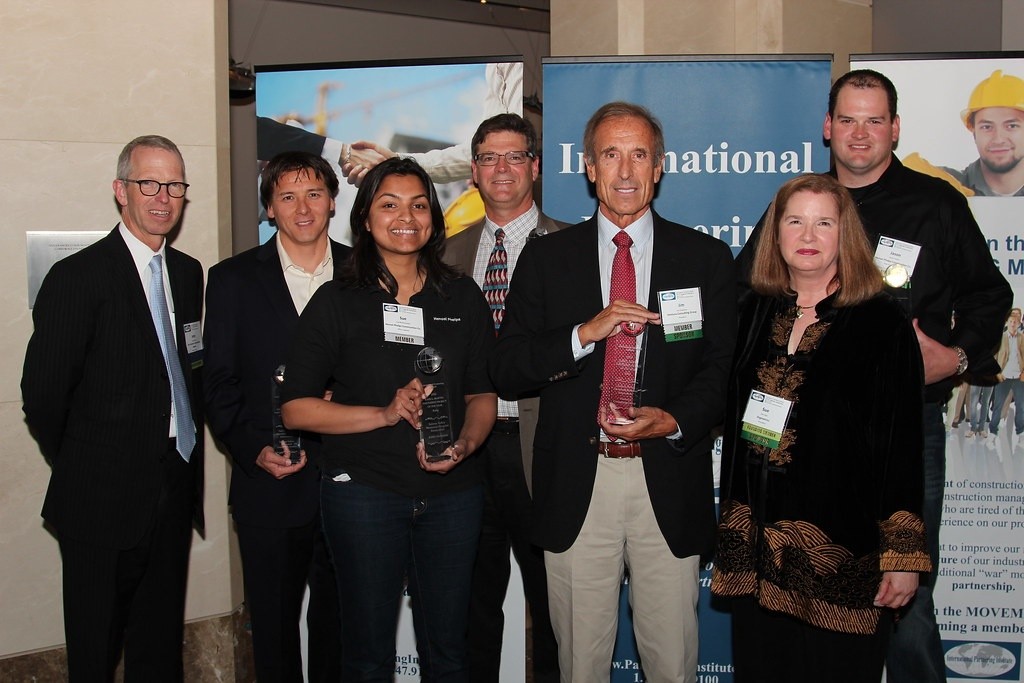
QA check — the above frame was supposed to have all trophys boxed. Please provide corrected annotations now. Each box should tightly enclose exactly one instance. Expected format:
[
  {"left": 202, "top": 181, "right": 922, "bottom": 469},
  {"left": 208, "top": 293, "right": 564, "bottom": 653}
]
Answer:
[
  {"left": 607, "top": 319, "right": 648, "bottom": 425},
  {"left": 884, "top": 263, "right": 915, "bottom": 323},
  {"left": 413, "top": 345, "right": 455, "bottom": 461},
  {"left": 272, "top": 364, "right": 302, "bottom": 463}
]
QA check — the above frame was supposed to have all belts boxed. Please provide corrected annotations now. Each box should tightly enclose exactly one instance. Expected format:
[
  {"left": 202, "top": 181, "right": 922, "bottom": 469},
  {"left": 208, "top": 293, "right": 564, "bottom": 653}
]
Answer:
[
  {"left": 493, "top": 417, "right": 519, "bottom": 434},
  {"left": 599, "top": 440, "right": 642, "bottom": 459}
]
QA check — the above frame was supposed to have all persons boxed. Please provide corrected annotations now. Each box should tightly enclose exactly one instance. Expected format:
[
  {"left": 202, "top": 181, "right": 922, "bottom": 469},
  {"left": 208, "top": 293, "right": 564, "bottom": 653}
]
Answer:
[
  {"left": 709, "top": 177, "right": 934, "bottom": 683},
  {"left": 945, "top": 307, "right": 1024, "bottom": 447},
  {"left": 256, "top": 115, "right": 387, "bottom": 171},
  {"left": 204, "top": 150, "right": 352, "bottom": 683},
  {"left": 342, "top": 62, "right": 523, "bottom": 188},
  {"left": 279, "top": 158, "right": 498, "bottom": 683},
  {"left": 441, "top": 114, "right": 574, "bottom": 683},
  {"left": 21, "top": 135, "right": 204, "bottom": 683},
  {"left": 903, "top": 70, "right": 1024, "bottom": 196},
  {"left": 733, "top": 68, "right": 1014, "bottom": 683},
  {"left": 494, "top": 102, "right": 742, "bottom": 683}
]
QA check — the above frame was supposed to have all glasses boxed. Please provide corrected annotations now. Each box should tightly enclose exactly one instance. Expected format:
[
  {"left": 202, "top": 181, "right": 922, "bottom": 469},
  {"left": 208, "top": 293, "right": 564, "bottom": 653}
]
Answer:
[
  {"left": 124, "top": 178, "right": 189, "bottom": 198},
  {"left": 474, "top": 151, "right": 534, "bottom": 166}
]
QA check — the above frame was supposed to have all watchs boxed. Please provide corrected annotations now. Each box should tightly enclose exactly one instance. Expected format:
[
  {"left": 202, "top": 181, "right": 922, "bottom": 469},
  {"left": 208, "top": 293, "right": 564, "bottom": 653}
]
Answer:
[{"left": 954, "top": 347, "right": 968, "bottom": 376}]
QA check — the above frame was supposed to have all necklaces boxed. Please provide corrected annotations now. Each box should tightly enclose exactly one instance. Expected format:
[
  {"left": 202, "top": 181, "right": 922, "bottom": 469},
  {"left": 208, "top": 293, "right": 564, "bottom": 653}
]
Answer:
[{"left": 796, "top": 305, "right": 815, "bottom": 319}]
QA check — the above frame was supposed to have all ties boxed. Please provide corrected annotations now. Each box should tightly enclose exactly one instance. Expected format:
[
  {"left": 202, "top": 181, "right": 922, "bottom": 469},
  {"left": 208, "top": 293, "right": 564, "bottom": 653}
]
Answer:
[
  {"left": 482, "top": 228, "right": 509, "bottom": 338},
  {"left": 148, "top": 253, "right": 197, "bottom": 463},
  {"left": 597, "top": 230, "right": 636, "bottom": 443}
]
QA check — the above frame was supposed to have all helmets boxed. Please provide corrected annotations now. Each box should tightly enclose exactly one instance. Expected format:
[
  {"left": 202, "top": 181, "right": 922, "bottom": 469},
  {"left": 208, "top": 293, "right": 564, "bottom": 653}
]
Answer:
[{"left": 961, "top": 71, "right": 1024, "bottom": 132}]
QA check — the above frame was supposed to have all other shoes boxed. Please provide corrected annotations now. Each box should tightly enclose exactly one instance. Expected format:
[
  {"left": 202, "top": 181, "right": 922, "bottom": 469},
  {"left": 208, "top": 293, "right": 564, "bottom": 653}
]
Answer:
[{"left": 944, "top": 413, "right": 1024, "bottom": 452}]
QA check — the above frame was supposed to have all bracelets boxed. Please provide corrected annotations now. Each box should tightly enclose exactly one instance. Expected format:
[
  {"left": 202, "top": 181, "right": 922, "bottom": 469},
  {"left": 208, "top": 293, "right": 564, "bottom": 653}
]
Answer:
[{"left": 345, "top": 145, "right": 351, "bottom": 164}]
